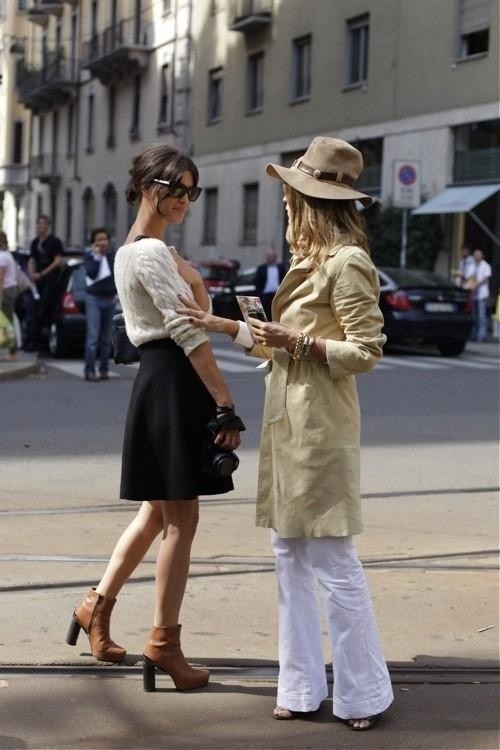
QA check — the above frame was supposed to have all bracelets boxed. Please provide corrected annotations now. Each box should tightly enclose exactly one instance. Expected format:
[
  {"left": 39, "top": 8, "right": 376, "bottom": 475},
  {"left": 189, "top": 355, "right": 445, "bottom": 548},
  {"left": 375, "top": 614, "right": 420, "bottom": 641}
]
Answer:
[
  {"left": 287, "top": 333, "right": 315, "bottom": 361},
  {"left": 39, "top": 270, "right": 43, "bottom": 278}
]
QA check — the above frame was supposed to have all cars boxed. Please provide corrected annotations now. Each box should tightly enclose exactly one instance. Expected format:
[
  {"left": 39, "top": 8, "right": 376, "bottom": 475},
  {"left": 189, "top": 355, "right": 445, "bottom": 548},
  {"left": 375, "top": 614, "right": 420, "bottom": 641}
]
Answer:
[{"left": 184, "top": 260, "right": 474, "bottom": 363}]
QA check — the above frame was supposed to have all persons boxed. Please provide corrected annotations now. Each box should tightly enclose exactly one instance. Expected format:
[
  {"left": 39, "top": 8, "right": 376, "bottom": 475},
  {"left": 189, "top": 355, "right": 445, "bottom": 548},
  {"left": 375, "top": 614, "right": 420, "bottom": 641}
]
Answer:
[
  {"left": 84, "top": 228, "right": 118, "bottom": 382},
  {"left": 0, "top": 232, "right": 20, "bottom": 361},
  {"left": 254, "top": 249, "right": 287, "bottom": 322},
  {"left": 451, "top": 244, "right": 475, "bottom": 287},
  {"left": 175, "top": 136, "right": 395, "bottom": 731},
  {"left": 67, "top": 145, "right": 244, "bottom": 691},
  {"left": 27, "top": 215, "right": 65, "bottom": 356},
  {"left": 467, "top": 249, "right": 493, "bottom": 342}
]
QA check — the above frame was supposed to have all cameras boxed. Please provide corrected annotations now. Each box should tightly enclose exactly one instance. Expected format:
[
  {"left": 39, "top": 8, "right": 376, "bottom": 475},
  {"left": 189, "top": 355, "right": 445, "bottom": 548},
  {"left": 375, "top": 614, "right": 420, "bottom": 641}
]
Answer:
[{"left": 194, "top": 440, "right": 239, "bottom": 480}]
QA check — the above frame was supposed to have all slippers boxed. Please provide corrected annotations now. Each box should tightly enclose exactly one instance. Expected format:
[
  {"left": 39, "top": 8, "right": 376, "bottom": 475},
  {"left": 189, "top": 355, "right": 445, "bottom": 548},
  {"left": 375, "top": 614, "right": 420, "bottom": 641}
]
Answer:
[
  {"left": 347, "top": 714, "right": 381, "bottom": 730},
  {"left": 273, "top": 704, "right": 305, "bottom": 720}
]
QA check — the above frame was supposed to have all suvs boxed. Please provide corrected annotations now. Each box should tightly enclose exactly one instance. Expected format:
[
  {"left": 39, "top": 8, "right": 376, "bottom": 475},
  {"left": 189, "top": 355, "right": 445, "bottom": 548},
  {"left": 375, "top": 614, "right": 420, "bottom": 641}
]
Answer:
[{"left": 13, "top": 247, "right": 124, "bottom": 359}]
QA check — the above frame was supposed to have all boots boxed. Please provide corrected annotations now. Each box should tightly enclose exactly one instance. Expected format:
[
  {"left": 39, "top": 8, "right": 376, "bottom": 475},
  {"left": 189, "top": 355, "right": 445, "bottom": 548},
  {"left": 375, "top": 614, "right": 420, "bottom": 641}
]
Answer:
[
  {"left": 66, "top": 586, "right": 127, "bottom": 662},
  {"left": 141, "top": 622, "right": 211, "bottom": 693}
]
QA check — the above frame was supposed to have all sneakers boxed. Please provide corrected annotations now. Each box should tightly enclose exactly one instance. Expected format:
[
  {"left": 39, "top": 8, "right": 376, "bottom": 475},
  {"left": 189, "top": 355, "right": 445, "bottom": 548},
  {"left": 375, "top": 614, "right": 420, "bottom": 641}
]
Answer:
[
  {"left": 85, "top": 373, "right": 99, "bottom": 381},
  {"left": 99, "top": 370, "right": 109, "bottom": 380}
]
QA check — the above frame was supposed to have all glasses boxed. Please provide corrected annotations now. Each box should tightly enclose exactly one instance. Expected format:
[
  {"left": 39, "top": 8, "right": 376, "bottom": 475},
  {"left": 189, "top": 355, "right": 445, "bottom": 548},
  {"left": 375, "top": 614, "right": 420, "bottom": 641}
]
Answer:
[{"left": 153, "top": 177, "right": 203, "bottom": 202}]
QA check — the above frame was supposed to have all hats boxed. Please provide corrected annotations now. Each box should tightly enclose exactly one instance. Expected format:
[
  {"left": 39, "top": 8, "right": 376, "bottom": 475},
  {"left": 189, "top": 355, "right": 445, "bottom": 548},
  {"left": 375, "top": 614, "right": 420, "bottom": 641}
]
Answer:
[{"left": 266, "top": 136, "right": 373, "bottom": 207}]
